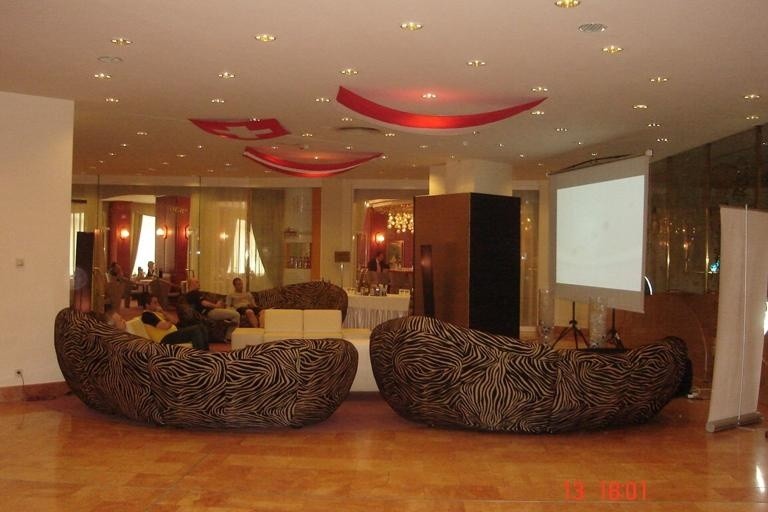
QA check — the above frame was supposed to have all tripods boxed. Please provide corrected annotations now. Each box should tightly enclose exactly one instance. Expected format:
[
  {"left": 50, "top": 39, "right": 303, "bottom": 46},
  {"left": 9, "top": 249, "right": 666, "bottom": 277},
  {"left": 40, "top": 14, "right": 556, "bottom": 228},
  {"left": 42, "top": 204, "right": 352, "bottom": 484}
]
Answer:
[
  {"left": 551, "top": 302, "right": 591, "bottom": 350},
  {"left": 590, "top": 308, "right": 626, "bottom": 351}
]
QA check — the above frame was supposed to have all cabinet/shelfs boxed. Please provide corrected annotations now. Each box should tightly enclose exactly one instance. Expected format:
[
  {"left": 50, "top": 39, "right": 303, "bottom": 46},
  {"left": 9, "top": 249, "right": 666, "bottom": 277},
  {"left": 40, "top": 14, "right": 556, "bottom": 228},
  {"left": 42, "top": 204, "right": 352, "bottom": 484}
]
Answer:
[
  {"left": 281, "top": 239, "right": 311, "bottom": 286},
  {"left": 412, "top": 191, "right": 521, "bottom": 340},
  {"left": 74, "top": 231, "right": 95, "bottom": 311}
]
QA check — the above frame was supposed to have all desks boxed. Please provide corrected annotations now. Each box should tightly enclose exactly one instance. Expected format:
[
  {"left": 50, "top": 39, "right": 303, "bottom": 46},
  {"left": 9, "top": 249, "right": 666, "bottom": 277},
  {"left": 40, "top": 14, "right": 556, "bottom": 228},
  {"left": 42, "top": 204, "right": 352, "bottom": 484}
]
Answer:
[
  {"left": 388, "top": 267, "right": 414, "bottom": 294},
  {"left": 342, "top": 293, "right": 410, "bottom": 328}
]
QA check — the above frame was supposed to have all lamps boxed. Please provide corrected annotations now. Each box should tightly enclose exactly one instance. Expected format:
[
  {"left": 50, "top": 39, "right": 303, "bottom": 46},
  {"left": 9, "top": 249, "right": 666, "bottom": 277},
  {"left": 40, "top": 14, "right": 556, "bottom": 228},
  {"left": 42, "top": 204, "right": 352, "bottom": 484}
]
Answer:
[
  {"left": 386, "top": 199, "right": 414, "bottom": 235},
  {"left": 156, "top": 225, "right": 167, "bottom": 239}
]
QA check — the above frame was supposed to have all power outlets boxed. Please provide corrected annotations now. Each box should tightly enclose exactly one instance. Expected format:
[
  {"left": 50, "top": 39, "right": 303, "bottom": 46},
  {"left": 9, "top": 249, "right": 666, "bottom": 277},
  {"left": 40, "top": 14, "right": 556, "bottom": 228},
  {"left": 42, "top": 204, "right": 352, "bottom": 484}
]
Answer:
[{"left": 15, "top": 368, "right": 23, "bottom": 376}]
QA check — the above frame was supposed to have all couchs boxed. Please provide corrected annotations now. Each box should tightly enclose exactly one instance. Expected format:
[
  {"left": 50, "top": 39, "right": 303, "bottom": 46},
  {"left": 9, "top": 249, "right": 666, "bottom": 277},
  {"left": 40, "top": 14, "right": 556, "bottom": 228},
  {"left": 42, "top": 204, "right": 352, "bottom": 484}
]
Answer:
[
  {"left": 231, "top": 309, "right": 379, "bottom": 392},
  {"left": 369, "top": 315, "right": 688, "bottom": 435},
  {"left": 54, "top": 308, "right": 359, "bottom": 431},
  {"left": 176, "top": 281, "right": 348, "bottom": 343},
  {"left": 126, "top": 316, "right": 192, "bottom": 348}
]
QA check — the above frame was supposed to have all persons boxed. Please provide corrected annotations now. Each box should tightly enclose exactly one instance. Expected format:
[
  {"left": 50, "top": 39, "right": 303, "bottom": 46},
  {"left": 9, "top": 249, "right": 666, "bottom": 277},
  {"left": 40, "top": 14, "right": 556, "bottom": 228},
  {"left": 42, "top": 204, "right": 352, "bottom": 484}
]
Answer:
[
  {"left": 365, "top": 251, "right": 403, "bottom": 273},
  {"left": 186, "top": 276, "right": 241, "bottom": 344},
  {"left": 225, "top": 277, "right": 259, "bottom": 328},
  {"left": 142, "top": 292, "right": 211, "bottom": 352},
  {"left": 97, "top": 260, "right": 157, "bottom": 283},
  {"left": 102, "top": 310, "right": 128, "bottom": 332}
]
